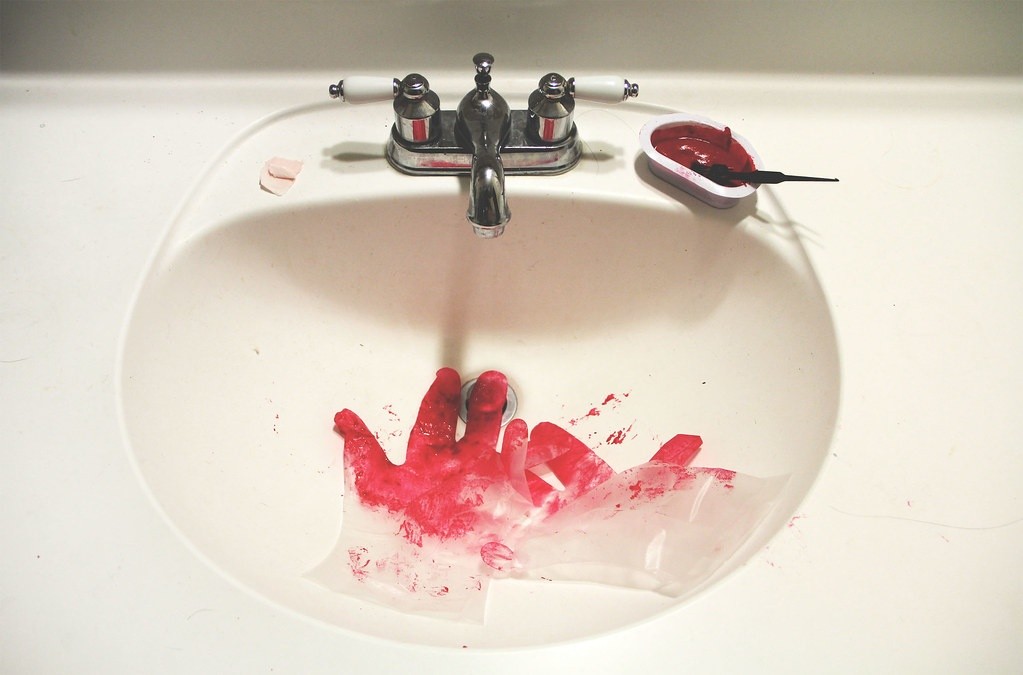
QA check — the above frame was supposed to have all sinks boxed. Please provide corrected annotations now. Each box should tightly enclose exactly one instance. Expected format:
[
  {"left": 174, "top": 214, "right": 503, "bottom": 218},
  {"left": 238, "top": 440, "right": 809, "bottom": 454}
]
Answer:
[{"left": 110, "top": 192, "right": 842, "bottom": 650}]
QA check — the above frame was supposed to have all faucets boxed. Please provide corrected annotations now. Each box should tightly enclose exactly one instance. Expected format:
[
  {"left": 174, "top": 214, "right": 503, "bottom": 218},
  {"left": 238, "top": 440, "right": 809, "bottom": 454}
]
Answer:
[{"left": 454, "top": 52, "right": 515, "bottom": 239}]
